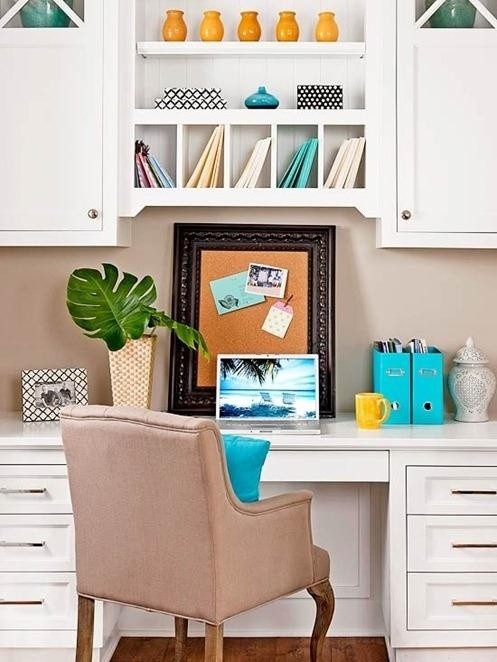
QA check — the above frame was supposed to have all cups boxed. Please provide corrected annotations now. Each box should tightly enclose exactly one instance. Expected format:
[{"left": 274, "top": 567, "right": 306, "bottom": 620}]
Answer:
[{"left": 354, "top": 391, "right": 390, "bottom": 432}]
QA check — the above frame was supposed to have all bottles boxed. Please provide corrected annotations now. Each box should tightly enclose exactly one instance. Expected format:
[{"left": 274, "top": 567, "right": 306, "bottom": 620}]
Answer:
[
  {"left": 243, "top": 86, "right": 280, "bottom": 109},
  {"left": 162, "top": 9, "right": 188, "bottom": 41},
  {"left": 315, "top": 12, "right": 339, "bottom": 41},
  {"left": 275, "top": 11, "right": 300, "bottom": 42},
  {"left": 238, "top": 11, "right": 262, "bottom": 42},
  {"left": 199, "top": 10, "right": 224, "bottom": 42}
]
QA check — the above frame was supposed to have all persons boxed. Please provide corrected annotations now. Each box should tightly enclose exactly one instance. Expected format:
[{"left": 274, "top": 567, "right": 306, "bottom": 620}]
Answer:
[
  {"left": 248, "top": 266, "right": 284, "bottom": 289},
  {"left": 30, "top": 384, "right": 73, "bottom": 408}
]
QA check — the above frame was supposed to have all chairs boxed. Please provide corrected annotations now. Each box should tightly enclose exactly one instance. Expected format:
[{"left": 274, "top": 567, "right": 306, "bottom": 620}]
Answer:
[{"left": 58, "top": 405, "right": 334, "bottom": 661}]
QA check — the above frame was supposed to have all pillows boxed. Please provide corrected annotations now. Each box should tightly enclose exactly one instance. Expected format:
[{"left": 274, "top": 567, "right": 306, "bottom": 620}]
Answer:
[{"left": 222, "top": 434, "right": 271, "bottom": 502}]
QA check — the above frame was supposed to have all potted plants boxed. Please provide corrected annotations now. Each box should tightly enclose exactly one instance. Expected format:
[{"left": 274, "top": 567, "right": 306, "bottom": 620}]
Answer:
[{"left": 66, "top": 263, "right": 211, "bottom": 410}]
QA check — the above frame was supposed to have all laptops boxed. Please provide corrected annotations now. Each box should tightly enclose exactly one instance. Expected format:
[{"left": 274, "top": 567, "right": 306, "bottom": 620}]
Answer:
[{"left": 214, "top": 354, "right": 323, "bottom": 435}]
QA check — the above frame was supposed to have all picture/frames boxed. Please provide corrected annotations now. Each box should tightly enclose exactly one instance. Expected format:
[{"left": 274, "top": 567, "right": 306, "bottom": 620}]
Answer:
[{"left": 169, "top": 224, "right": 336, "bottom": 418}]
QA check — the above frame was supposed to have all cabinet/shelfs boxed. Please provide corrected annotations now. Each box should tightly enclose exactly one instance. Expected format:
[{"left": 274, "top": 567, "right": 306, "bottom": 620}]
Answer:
[
  {"left": 2, "top": 3, "right": 120, "bottom": 247},
  {"left": 375, "top": 0, "right": 497, "bottom": 248},
  {"left": 0, "top": 411, "right": 497, "bottom": 662},
  {"left": 120, "top": 2, "right": 383, "bottom": 220}
]
